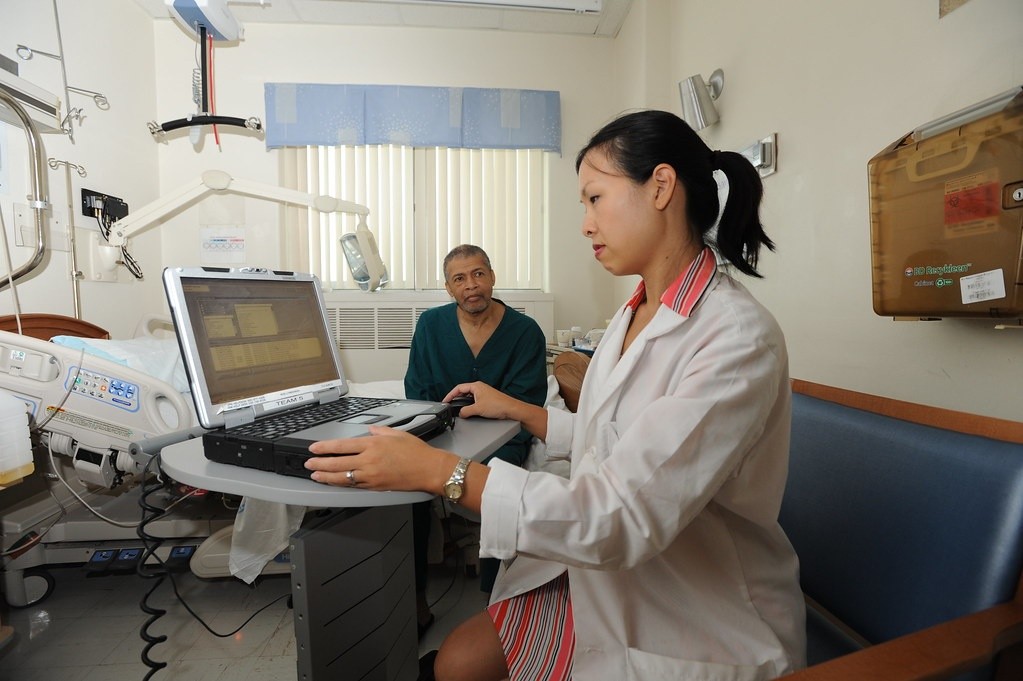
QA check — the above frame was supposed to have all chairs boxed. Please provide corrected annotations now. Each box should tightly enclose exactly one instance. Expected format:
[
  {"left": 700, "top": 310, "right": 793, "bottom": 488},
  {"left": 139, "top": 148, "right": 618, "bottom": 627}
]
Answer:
[{"left": 774, "top": 378, "right": 1023, "bottom": 681}]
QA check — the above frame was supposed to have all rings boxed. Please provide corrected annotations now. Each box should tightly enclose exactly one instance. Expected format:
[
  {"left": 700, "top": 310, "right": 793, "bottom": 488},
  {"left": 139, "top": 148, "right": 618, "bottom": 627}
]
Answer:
[{"left": 347, "top": 471, "right": 355, "bottom": 485}]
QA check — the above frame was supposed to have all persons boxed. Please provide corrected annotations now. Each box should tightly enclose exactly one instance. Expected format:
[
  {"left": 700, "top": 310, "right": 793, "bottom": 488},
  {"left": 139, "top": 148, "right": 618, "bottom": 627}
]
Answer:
[
  {"left": 403, "top": 244, "right": 548, "bottom": 642},
  {"left": 307, "top": 110, "right": 806, "bottom": 681}
]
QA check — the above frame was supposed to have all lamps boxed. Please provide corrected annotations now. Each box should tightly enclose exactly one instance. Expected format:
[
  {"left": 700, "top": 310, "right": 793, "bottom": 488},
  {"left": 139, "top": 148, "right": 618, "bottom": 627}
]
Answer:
[
  {"left": 93, "top": 169, "right": 390, "bottom": 294},
  {"left": 677, "top": 67, "right": 725, "bottom": 131}
]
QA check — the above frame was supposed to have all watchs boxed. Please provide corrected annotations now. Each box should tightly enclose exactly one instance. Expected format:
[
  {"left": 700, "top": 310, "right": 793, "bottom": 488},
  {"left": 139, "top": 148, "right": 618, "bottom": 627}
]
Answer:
[{"left": 443, "top": 457, "right": 472, "bottom": 504}]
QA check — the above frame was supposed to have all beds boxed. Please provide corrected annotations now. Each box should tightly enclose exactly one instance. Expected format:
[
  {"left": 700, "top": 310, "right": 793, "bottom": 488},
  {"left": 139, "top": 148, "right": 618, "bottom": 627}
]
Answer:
[{"left": 0, "top": 317, "right": 293, "bottom": 609}]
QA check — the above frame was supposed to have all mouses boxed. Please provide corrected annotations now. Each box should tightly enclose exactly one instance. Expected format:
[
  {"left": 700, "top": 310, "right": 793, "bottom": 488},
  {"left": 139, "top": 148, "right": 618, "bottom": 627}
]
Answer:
[{"left": 447, "top": 396, "right": 483, "bottom": 418}]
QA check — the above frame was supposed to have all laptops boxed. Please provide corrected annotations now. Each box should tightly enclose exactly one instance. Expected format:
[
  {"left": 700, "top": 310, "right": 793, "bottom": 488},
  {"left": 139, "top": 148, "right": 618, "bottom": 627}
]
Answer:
[{"left": 162, "top": 266, "right": 456, "bottom": 480}]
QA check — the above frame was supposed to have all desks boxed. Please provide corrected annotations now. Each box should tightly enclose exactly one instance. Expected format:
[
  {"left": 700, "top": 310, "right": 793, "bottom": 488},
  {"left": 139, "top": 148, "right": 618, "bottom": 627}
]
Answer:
[{"left": 161, "top": 410, "right": 523, "bottom": 681}]
